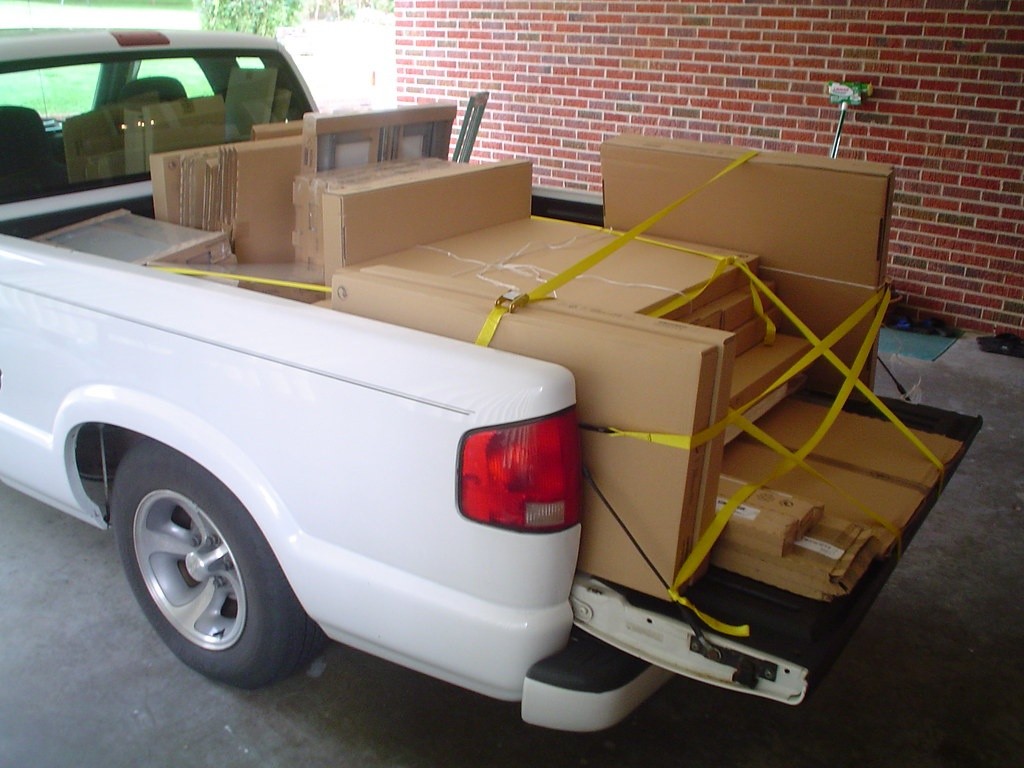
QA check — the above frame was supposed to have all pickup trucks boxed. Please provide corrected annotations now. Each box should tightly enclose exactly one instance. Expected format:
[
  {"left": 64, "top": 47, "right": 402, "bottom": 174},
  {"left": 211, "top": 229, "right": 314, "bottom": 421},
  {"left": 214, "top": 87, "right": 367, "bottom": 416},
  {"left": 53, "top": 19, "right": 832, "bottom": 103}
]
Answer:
[{"left": 0, "top": 27, "right": 982, "bottom": 732}]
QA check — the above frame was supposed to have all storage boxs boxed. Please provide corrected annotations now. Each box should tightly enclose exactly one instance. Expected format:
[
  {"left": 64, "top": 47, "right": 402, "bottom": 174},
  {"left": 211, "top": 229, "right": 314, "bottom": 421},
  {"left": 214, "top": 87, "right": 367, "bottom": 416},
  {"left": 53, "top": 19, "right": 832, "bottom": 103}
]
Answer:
[
  {"left": 123, "top": 95, "right": 226, "bottom": 179},
  {"left": 294, "top": 133, "right": 965, "bottom": 601}
]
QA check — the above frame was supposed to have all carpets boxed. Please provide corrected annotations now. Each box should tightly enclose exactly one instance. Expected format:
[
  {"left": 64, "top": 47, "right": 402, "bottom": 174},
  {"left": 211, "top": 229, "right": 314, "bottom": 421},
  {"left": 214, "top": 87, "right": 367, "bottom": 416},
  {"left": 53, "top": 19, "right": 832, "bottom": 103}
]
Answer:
[{"left": 879, "top": 325, "right": 957, "bottom": 362}]
